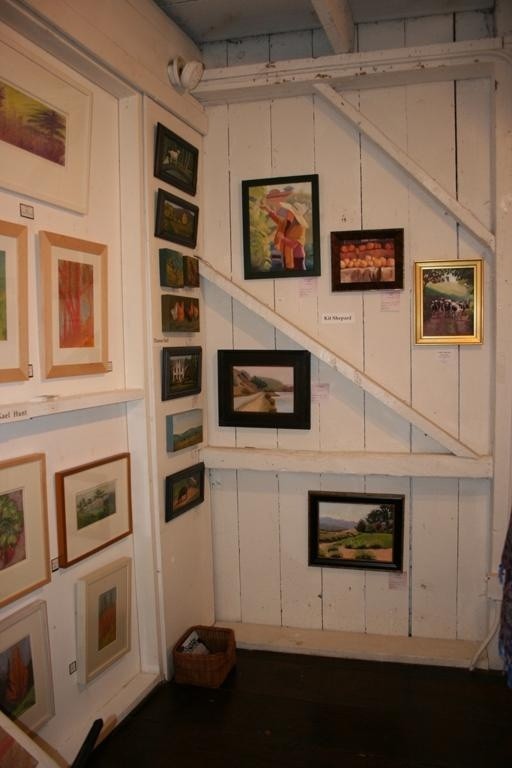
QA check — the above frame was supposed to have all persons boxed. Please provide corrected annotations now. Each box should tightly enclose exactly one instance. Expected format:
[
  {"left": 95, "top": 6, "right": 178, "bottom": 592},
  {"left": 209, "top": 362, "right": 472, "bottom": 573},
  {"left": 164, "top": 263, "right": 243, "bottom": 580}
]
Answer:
[{"left": 259, "top": 201, "right": 311, "bottom": 272}]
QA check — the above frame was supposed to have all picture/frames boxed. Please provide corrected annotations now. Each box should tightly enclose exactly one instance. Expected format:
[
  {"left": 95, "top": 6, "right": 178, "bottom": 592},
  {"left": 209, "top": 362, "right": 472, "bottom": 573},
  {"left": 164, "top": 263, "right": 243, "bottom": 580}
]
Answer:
[
  {"left": 308, "top": 490, "right": 405, "bottom": 572},
  {"left": 165, "top": 462, "right": 205, "bottom": 523},
  {"left": 217, "top": 349, "right": 312, "bottom": 430},
  {"left": 414, "top": 258, "right": 484, "bottom": 346},
  {"left": 330, "top": 228, "right": 404, "bottom": 292},
  {"left": 241, "top": 173, "right": 321, "bottom": 280},
  {"left": 0, "top": 33, "right": 109, "bottom": 383},
  {"left": 0, "top": 452, "right": 133, "bottom": 734},
  {"left": 153, "top": 121, "right": 199, "bottom": 249},
  {"left": 162, "top": 346, "right": 202, "bottom": 401}
]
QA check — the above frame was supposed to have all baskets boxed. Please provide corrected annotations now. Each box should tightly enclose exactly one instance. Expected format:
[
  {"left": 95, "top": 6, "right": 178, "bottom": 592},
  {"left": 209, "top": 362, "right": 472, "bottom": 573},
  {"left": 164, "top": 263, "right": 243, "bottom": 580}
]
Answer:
[{"left": 172, "top": 626, "right": 236, "bottom": 689}]
